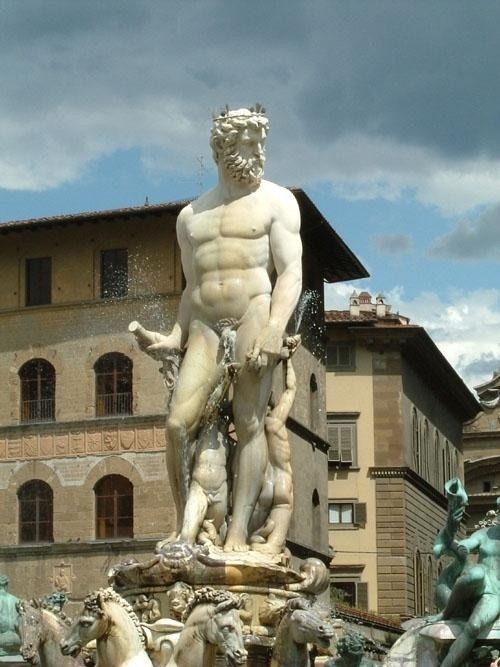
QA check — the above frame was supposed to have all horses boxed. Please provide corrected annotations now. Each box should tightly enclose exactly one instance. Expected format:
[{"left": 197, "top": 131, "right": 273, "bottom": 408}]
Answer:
[
  {"left": 59, "top": 584, "right": 153, "bottom": 667},
  {"left": 13, "top": 595, "right": 90, "bottom": 667},
  {"left": 269, "top": 598, "right": 335, "bottom": 667},
  {"left": 167, "top": 587, "right": 249, "bottom": 667}
]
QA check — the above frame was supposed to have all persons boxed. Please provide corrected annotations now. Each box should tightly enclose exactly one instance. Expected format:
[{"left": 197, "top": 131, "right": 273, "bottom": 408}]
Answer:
[
  {"left": 252, "top": 335, "right": 301, "bottom": 556},
  {"left": 425, "top": 478, "right": 500, "bottom": 667},
  {"left": 174, "top": 404, "right": 229, "bottom": 549},
  {"left": 141, "top": 107, "right": 303, "bottom": 552}
]
